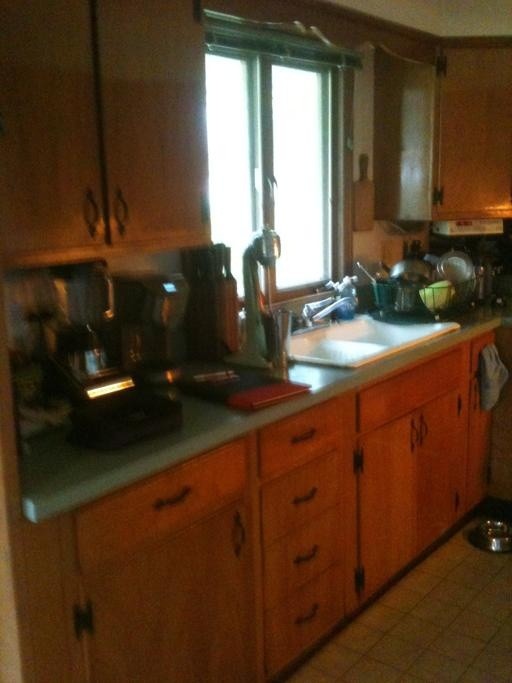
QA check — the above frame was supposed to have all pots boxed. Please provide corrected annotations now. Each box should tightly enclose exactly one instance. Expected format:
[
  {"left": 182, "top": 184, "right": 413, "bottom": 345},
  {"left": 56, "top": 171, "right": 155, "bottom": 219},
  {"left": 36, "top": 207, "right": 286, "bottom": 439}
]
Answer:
[{"left": 356, "top": 261, "right": 417, "bottom": 313}]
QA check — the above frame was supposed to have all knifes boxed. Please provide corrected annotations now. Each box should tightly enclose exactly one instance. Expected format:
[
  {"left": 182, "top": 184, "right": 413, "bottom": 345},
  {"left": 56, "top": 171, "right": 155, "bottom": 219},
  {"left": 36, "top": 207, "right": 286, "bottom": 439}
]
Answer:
[{"left": 179, "top": 244, "right": 232, "bottom": 281}]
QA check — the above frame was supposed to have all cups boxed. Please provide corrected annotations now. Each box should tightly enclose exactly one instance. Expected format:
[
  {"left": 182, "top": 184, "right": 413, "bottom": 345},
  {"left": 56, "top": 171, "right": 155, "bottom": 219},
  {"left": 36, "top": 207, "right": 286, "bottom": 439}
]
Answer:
[{"left": 419, "top": 280, "right": 456, "bottom": 315}]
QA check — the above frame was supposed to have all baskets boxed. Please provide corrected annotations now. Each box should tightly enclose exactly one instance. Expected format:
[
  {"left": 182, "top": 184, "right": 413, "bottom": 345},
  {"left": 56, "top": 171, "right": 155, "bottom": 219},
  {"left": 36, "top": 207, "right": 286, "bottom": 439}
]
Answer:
[{"left": 369, "top": 272, "right": 483, "bottom": 312}]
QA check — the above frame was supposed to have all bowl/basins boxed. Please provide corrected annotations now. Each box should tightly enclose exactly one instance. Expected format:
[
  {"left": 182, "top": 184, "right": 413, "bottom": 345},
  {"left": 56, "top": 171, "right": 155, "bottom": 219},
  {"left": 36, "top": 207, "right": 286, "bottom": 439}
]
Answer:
[
  {"left": 468, "top": 519, "right": 512, "bottom": 552},
  {"left": 390, "top": 257, "right": 433, "bottom": 283}
]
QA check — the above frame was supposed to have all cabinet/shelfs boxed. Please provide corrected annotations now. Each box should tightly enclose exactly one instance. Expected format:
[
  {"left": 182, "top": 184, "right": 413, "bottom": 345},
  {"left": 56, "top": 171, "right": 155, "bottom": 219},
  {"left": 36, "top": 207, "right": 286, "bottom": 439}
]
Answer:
[
  {"left": 0, "top": 0, "right": 211, "bottom": 271},
  {"left": 354, "top": 340, "right": 472, "bottom": 601},
  {"left": 374, "top": 42, "right": 512, "bottom": 222},
  {"left": 257, "top": 390, "right": 358, "bottom": 678},
  {"left": 72, "top": 433, "right": 267, "bottom": 683},
  {"left": 468, "top": 328, "right": 492, "bottom": 510}
]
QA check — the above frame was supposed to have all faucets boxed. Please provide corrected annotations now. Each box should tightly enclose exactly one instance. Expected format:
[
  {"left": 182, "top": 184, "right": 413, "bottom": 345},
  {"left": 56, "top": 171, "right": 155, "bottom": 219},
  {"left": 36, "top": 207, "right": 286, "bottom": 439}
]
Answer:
[{"left": 300, "top": 296, "right": 351, "bottom": 328}]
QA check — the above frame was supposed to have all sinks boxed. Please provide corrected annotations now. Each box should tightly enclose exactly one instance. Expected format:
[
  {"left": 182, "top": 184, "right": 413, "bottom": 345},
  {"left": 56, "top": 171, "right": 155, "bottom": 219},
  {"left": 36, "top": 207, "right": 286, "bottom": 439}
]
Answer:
[
  {"left": 302, "top": 313, "right": 462, "bottom": 350},
  {"left": 286, "top": 336, "right": 388, "bottom": 368}
]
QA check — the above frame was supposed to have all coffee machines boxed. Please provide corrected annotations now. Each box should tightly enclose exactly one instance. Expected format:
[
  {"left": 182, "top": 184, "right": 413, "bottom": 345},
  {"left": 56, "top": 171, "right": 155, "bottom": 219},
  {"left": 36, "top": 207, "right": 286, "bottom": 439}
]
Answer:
[{"left": 27, "top": 257, "right": 183, "bottom": 454}]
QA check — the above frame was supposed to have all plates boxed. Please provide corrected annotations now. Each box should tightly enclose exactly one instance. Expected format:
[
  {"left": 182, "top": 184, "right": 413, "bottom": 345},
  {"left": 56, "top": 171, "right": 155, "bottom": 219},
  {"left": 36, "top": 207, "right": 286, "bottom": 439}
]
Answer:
[{"left": 437, "top": 251, "right": 474, "bottom": 285}]
